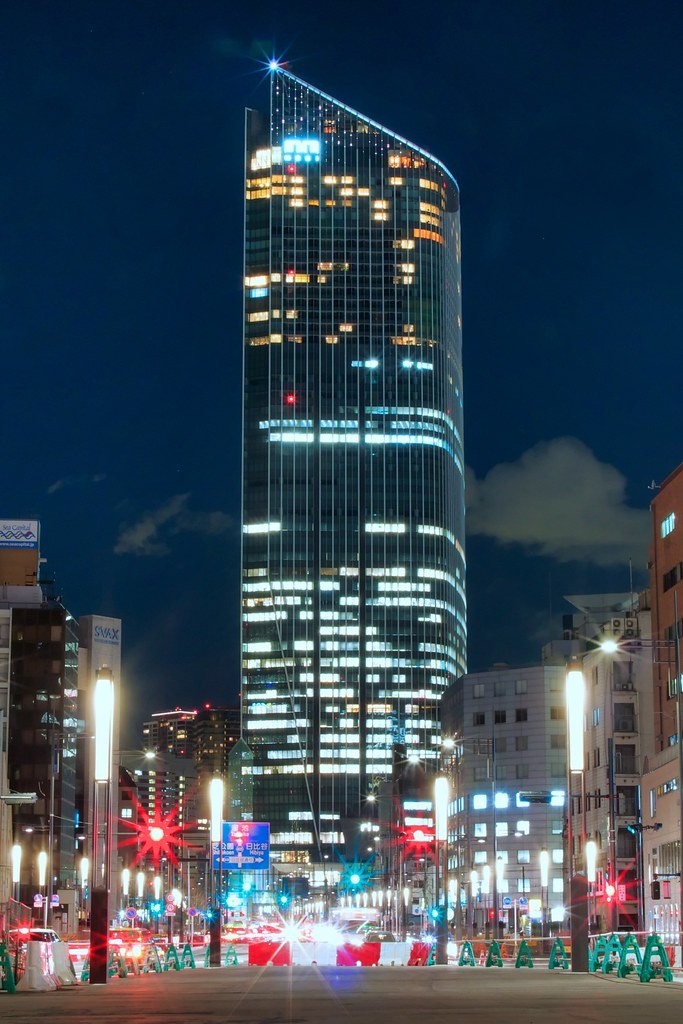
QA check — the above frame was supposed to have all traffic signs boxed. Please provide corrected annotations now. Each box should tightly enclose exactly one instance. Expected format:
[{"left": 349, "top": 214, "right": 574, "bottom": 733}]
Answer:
[{"left": 214, "top": 821, "right": 270, "bottom": 870}]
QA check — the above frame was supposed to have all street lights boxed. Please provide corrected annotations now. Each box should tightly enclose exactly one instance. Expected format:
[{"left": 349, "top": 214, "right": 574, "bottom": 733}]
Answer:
[
  {"left": 90, "top": 666, "right": 116, "bottom": 986},
  {"left": 46, "top": 734, "right": 95, "bottom": 931},
  {"left": 433, "top": 776, "right": 449, "bottom": 965},
  {"left": 442, "top": 735, "right": 499, "bottom": 945},
  {"left": 540, "top": 847, "right": 550, "bottom": 954},
  {"left": 564, "top": 655, "right": 590, "bottom": 975},
  {"left": 209, "top": 778, "right": 224, "bottom": 968},
  {"left": 517, "top": 791, "right": 617, "bottom": 959},
  {"left": 372, "top": 854, "right": 504, "bottom": 950},
  {"left": 10, "top": 846, "right": 161, "bottom": 936},
  {"left": 602, "top": 638, "right": 618, "bottom": 936},
  {"left": 365, "top": 793, "right": 403, "bottom": 893}
]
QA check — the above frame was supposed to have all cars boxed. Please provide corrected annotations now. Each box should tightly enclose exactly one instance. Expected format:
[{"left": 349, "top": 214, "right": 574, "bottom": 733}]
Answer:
[
  {"left": 223, "top": 920, "right": 379, "bottom": 944},
  {"left": 4, "top": 929, "right": 62, "bottom": 955}
]
